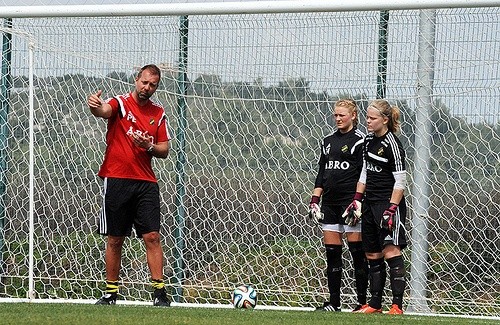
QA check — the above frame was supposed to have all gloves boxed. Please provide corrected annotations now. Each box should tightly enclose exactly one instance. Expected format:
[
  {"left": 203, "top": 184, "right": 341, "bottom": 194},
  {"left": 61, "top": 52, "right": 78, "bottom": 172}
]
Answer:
[
  {"left": 380, "top": 203, "right": 398, "bottom": 232},
  {"left": 308, "top": 196, "right": 321, "bottom": 219},
  {"left": 342, "top": 192, "right": 365, "bottom": 226}
]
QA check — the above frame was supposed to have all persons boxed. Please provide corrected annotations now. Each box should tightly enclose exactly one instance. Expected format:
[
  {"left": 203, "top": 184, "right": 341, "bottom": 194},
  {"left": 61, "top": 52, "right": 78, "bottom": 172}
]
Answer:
[
  {"left": 88, "top": 65, "right": 171, "bottom": 306},
  {"left": 342, "top": 99, "right": 407, "bottom": 315},
  {"left": 308, "top": 99, "right": 367, "bottom": 312}
]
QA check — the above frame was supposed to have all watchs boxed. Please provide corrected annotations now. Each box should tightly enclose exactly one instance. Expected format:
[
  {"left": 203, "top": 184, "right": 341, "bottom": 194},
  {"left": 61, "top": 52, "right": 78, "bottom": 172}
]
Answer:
[{"left": 147, "top": 145, "right": 154, "bottom": 153}]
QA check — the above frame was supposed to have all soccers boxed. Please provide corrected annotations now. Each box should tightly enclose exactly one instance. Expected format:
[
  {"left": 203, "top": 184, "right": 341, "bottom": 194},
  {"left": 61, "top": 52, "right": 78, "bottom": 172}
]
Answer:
[{"left": 232, "top": 284, "right": 257, "bottom": 310}]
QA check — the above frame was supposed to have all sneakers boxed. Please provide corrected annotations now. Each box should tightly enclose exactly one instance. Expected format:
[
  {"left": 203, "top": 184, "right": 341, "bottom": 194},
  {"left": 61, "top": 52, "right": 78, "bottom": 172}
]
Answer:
[
  {"left": 154, "top": 288, "right": 170, "bottom": 306},
  {"left": 316, "top": 303, "right": 342, "bottom": 312},
  {"left": 96, "top": 293, "right": 116, "bottom": 305},
  {"left": 352, "top": 304, "right": 368, "bottom": 313},
  {"left": 361, "top": 306, "right": 382, "bottom": 314},
  {"left": 387, "top": 304, "right": 403, "bottom": 314}
]
